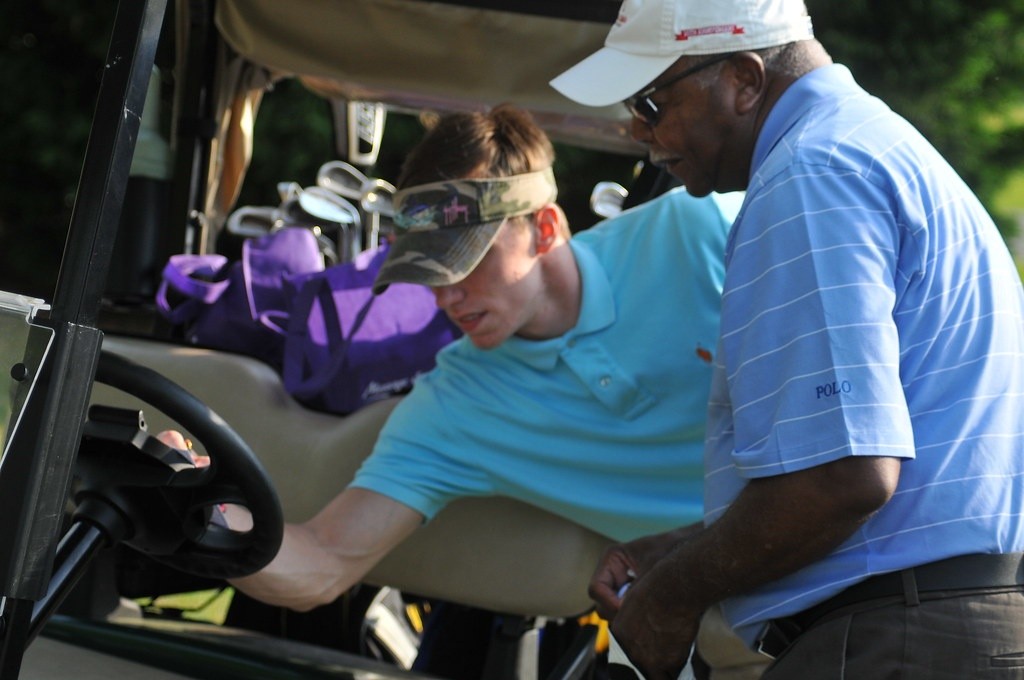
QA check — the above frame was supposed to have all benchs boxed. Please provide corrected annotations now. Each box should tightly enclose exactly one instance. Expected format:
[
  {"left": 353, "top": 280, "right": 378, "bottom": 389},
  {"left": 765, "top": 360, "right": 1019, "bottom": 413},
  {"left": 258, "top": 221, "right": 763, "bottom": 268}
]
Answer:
[{"left": 36, "top": 335, "right": 614, "bottom": 680}]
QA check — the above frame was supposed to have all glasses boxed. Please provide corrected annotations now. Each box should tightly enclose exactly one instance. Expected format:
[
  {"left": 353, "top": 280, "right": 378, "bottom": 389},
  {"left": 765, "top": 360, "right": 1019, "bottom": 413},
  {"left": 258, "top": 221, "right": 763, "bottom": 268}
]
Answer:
[{"left": 623, "top": 53, "right": 732, "bottom": 126}]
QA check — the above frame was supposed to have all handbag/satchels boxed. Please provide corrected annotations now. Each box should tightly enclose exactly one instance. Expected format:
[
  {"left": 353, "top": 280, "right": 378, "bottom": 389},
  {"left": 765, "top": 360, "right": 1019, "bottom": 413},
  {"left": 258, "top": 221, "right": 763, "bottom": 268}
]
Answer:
[
  {"left": 153, "top": 225, "right": 329, "bottom": 357},
  {"left": 283, "top": 243, "right": 457, "bottom": 418}
]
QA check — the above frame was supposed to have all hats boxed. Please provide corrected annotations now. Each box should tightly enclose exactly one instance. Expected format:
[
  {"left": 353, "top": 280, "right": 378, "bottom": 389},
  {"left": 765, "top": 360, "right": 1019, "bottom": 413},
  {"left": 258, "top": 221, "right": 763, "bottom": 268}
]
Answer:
[
  {"left": 371, "top": 168, "right": 558, "bottom": 295},
  {"left": 548, "top": 0, "right": 818, "bottom": 108}
]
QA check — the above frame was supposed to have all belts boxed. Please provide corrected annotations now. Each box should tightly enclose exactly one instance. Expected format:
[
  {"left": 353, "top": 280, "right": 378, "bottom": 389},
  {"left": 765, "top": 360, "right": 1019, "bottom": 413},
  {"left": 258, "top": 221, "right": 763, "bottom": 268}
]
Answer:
[{"left": 756, "top": 552, "right": 1024, "bottom": 660}]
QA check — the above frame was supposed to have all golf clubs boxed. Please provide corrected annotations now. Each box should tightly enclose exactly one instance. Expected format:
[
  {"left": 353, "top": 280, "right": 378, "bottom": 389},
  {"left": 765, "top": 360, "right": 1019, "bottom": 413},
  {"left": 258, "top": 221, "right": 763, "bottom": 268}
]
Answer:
[
  {"left": 588, "top": 179, "right": 629, "bottom": 219},
  {"left": 226, "top": 99, "right": 400, "bottom": 267}
]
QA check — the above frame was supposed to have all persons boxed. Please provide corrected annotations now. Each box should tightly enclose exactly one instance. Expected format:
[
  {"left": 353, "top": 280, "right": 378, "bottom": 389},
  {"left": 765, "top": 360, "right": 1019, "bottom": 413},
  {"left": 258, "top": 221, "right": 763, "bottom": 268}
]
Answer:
[
  {"left": 550, "top": 0, "right": 1024, "bottom": 680},
  {"left": 155, "top": 102, "right": 748, "bottom": 611}
]
